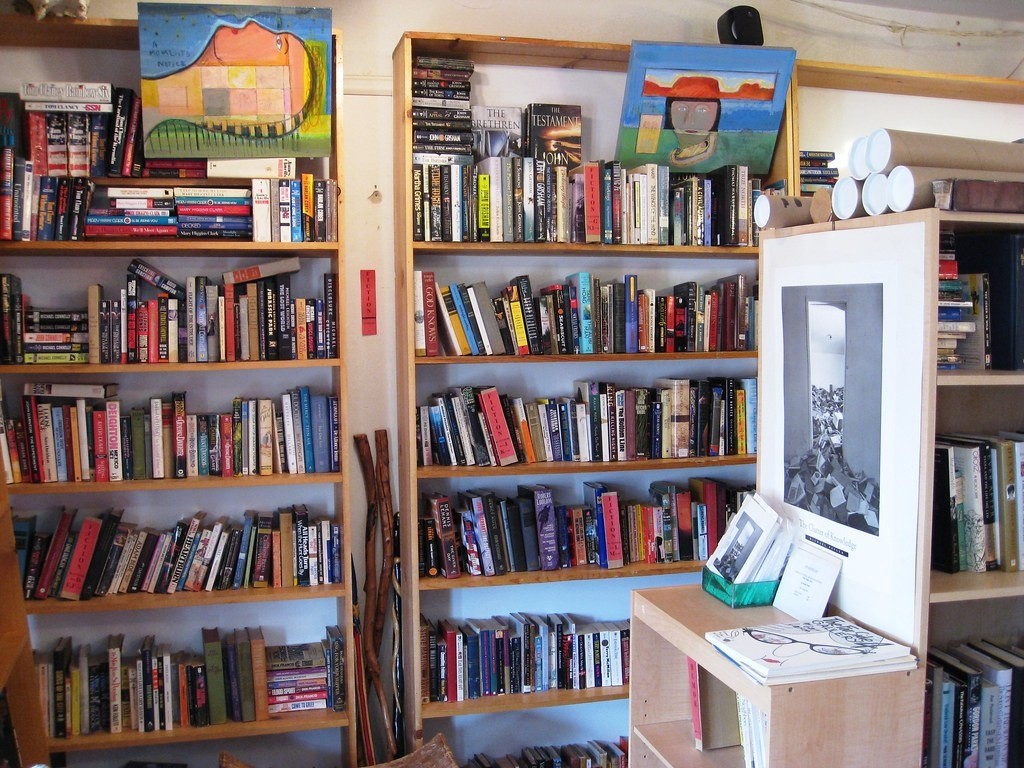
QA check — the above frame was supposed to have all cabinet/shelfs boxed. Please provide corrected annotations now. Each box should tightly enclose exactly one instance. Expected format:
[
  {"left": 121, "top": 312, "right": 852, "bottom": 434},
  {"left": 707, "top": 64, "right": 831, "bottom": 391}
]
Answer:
[
  {"left": 754, "top": 206, "right": 1024, "bottom": 768},
  {"left": 1, "top": 1, "right": 359, "bottom": 768},
  {"left": 618, "top": 569, "right": 917, "bottom": 768},
  {"left": 392, "top": 27, "right": 1024, "bottom": 768}
]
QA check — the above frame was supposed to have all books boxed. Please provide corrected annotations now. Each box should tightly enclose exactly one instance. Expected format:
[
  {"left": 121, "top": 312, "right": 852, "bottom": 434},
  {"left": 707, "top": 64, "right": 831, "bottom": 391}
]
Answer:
[{"left": 0, "top": 52, "right": 1024, "bottom": 768}]
